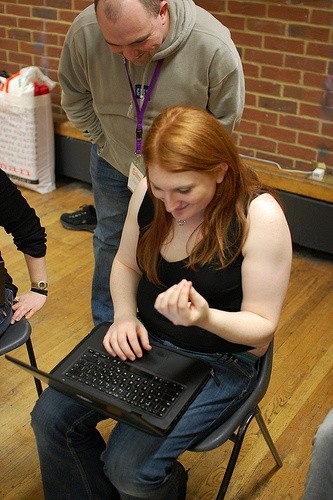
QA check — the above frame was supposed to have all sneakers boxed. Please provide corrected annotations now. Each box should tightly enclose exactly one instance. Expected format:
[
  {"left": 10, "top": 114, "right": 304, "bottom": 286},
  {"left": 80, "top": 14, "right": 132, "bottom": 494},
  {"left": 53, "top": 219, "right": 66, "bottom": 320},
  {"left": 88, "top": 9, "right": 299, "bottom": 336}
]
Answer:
[{"left": 61, "top": 205, "right": 97, "bottom": 230}]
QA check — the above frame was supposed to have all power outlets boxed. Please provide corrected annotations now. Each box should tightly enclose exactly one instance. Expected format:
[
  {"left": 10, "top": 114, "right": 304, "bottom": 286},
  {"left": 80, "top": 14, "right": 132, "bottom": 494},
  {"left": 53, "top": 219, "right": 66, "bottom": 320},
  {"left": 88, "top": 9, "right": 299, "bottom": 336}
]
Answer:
[{"left": 313, "top": 163, "right": 327, "bottom": 174}]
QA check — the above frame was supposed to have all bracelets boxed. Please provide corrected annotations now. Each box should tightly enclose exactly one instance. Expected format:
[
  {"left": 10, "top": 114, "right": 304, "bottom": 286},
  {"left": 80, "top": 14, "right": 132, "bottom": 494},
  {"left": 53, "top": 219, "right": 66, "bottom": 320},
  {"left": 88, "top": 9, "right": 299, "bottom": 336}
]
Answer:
[{"left": 31, "top": 288, "right": 48, "bottom": 296}]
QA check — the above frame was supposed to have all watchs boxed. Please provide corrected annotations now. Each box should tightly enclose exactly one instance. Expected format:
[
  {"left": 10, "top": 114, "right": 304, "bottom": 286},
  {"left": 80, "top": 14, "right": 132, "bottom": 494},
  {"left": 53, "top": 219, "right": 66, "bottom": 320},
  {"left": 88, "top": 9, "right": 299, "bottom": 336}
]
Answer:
[{"left": 32, "top": 279, "right": 48, "bottom": 290}]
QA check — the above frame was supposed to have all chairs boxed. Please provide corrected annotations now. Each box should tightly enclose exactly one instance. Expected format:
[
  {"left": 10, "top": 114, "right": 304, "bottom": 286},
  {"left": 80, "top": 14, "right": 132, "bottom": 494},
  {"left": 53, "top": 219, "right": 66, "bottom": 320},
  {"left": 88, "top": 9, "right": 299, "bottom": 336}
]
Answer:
[{"left": 0, "top": 316, "right": 282, "bottom": 500}]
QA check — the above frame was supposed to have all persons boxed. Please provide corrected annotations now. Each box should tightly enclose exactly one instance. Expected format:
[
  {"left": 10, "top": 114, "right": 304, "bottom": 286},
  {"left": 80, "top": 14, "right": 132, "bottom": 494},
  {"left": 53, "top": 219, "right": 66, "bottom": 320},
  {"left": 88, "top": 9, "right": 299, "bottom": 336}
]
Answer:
[
  {"left": 30, "top": 105, "right": 293, "bottom": 500},
  {"left": 58, "top": 0, "right": 245, "bottom": 327},
  {"left": 0, "top": 168, "right": 48, "bottom": 336}
]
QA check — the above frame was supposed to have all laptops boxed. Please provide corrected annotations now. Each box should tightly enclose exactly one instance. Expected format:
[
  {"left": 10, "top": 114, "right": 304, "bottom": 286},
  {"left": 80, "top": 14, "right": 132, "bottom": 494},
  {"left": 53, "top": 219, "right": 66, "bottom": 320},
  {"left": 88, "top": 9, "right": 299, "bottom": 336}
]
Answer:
[{"left": 5, "top": 321, "right": 210, "bottom": 437}]
